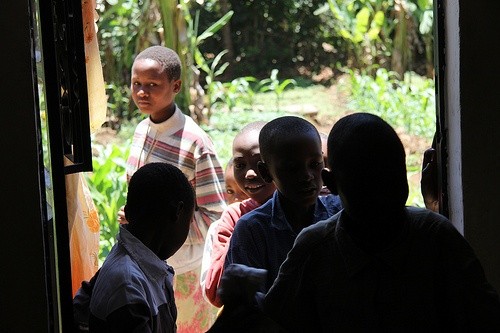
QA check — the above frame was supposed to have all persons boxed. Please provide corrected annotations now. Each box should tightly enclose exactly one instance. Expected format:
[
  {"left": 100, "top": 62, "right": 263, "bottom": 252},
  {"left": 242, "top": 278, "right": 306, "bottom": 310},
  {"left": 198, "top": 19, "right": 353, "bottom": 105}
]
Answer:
[
  {"left": 117, "top": 46, "right": 229, "bottom": 333},
  {"left": 205, "top": 120, "right": 277, "bottom": 308},
  {"left": 90, "top": 162, "right": 196, "bottom": 333},
  {"left": 216, "top": 116, "right": 342, "bottom": 333},
  {"left": 260, "top": 113, "right": 500, "bottom": 333},
  {"left": 200, "top": 157, "right": 249, "bottom": 305}
]
